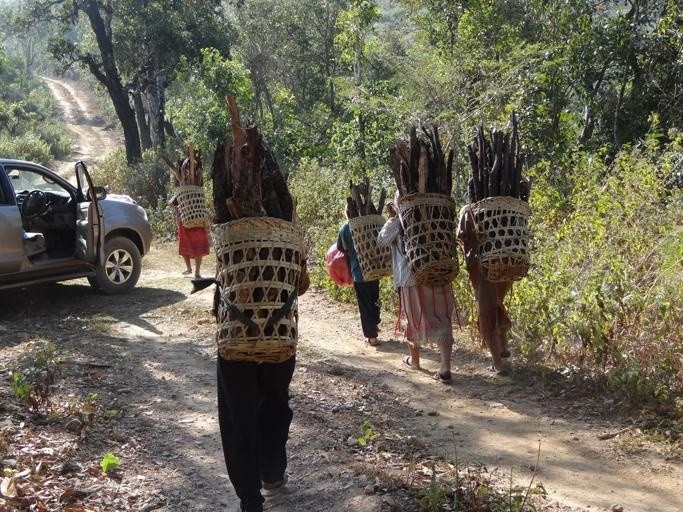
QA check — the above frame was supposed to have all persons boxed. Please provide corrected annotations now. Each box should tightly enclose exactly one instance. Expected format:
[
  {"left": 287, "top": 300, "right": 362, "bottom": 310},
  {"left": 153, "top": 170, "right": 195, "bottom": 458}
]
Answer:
[
  {"left": 208, "top": 247, "right": 312, "bottom": 512},
  {"left": 374, "top": 187, "right": 456, "bottom": 386},
  {"left": 336, "top": 198, "right": 382, "bottom": 345},
  {"left": 384, "top": 201, "right": 396, "bottom": 220},
  {"left": 169, "top": 177, "right": 212, "bottom": 279},
  {"left": 453, "top": 189, "right": 514, "bottom": 369}
]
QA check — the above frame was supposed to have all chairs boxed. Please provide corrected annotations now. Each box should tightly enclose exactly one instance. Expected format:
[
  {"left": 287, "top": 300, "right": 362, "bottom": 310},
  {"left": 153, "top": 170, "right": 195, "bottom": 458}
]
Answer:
[{"left": 24, "top": 230, "right": 47, "bottom": 256}]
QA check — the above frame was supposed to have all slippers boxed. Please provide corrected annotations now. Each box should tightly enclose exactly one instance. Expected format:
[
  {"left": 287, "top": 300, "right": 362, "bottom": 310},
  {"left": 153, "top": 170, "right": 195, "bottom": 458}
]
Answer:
[
  {"left": 432, "top": 371, "right": 452, "bottom": 383},
  {"left": 403, "top": 357, "right": 412, "bottom": 366},
  {"left": 261, "top": 472, "right": 288, "bottom": 497},
  {"left": 364, "top": 338, "right": 381, "bottom": 345},
  {"left": 487, "top": 351, "right": 510, "bottom": 374},
  {"left": 182, "top": 268, "right": 201, "bottom": 278}
]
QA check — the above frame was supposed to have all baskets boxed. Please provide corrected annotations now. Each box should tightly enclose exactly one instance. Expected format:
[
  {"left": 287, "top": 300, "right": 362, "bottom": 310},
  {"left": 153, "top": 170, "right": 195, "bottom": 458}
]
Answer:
[
  {"left": 174, "top": 184, "right": 207, "bottom": 228},
  {"left": 401, "top": 193, "right": 460, "bottom": 288},
  {"left": 210, "top": 216, "right": 303, "bottom": 363},
  {"left": 469, "top": 196, "right": 530, "bottom": 283},
  {"left": 348, "top": 214, "right": 393, "bottom": 282}
]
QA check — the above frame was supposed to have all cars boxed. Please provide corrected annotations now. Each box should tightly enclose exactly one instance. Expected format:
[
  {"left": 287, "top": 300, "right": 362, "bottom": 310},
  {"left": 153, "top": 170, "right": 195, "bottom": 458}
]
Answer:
[{"left": 0, "top": 159, "right": 154, "bottom": 296}]
